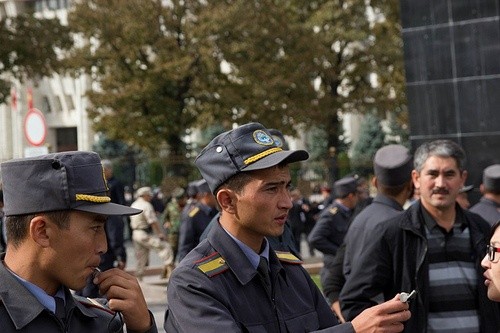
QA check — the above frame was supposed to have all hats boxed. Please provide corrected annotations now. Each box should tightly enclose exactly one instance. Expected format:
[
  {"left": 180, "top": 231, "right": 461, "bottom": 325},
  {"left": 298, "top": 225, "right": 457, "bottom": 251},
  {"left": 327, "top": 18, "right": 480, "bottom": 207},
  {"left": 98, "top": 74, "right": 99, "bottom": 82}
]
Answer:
[
  {"left": 0, "top": 151, "right": 143, "bottom": 216},
  {"left": 187, "top": 179, "right": 209, "bottom": 196},
  {"left": 172, "top": 188, "right": 185, "bottom": 199},
  {"left": 483, "top": 164, "right": 500, "bottom": 189},
  {"left": 137, "top": 186, "right": 153, "bottom": 196},
  {"left": 193, "top": 122, "right": 309, "bottom": 195},
  {"left": 373, "top": 144, "right": 414, "bottom": 186},
  {"left": 265, "top": 128, "right": 290, "bottom": 152},
  {"left": 334, "top": 176, "right": 358, "bottom": 197}
]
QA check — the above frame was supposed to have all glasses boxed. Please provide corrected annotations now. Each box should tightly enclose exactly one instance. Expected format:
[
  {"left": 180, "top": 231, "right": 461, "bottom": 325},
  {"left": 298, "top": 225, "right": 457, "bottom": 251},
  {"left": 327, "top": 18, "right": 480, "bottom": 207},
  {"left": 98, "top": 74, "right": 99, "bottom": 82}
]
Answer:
[{"left": 483, "top": 244, "right": 500, "bottom": 261}]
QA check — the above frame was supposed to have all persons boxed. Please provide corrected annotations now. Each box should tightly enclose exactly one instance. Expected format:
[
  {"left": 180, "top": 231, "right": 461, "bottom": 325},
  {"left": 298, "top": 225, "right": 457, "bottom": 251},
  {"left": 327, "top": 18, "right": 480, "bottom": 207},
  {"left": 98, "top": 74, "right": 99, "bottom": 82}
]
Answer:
[
  {"left": 163, "top": 121, "right": 411, "bottom": 333},
  {"left": 76, "top": 159, "right": 218, "bottom": 298},
  {"left": 0, "top": 152, "right": 158, "bottom": 333},
  {"left": 456, "top": 164, "right": 500, "bottom": 227},
  {"left": 338, "top": 140, "right": 500, "bottom": 333},
  {"left": 481, "top": 221, "right": 500, "bottom": 302},
  {"left": 269, "top": 129, "right": 421, "bottom": 325}
]
